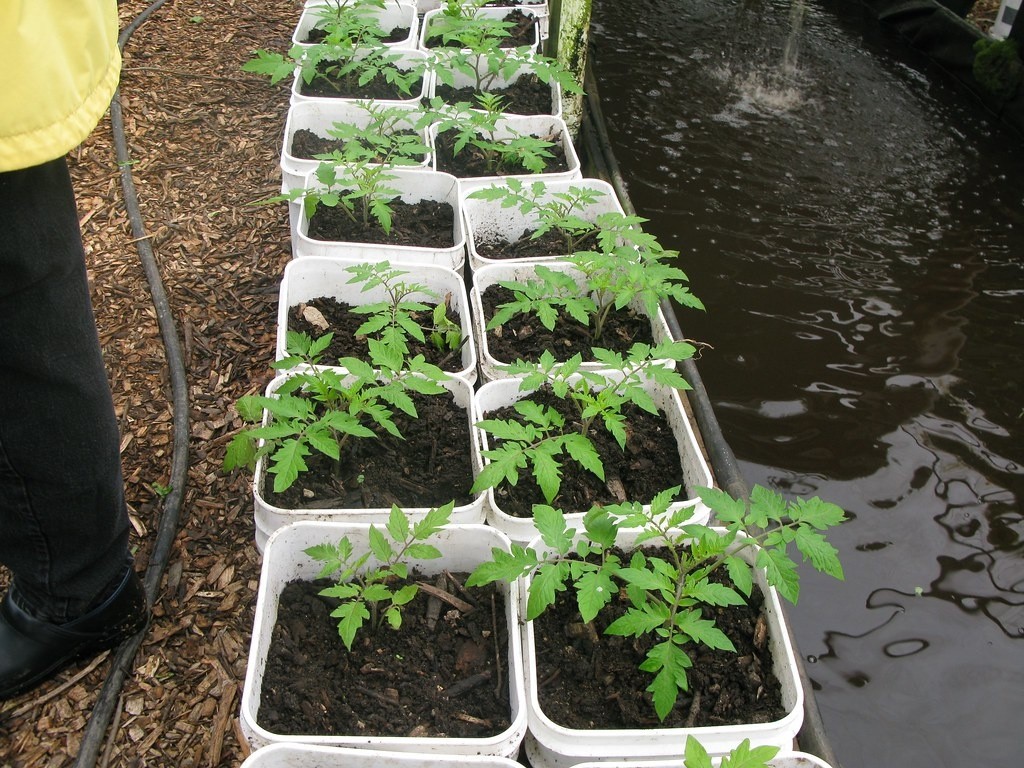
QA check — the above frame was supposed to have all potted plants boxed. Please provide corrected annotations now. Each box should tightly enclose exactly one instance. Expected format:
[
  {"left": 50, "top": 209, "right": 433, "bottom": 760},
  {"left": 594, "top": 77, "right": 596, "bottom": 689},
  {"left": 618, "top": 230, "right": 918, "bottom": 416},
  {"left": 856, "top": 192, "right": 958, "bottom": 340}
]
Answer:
[{"left": 241, "top": 0, "right": 831, "bottom": 768}]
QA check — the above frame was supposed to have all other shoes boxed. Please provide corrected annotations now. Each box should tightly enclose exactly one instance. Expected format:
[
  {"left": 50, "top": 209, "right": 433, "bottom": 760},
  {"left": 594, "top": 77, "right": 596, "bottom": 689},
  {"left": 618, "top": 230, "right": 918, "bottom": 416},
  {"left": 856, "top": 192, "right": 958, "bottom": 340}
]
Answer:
[{"left": 0, "top": 550, "right": 148, "bottom": 700}]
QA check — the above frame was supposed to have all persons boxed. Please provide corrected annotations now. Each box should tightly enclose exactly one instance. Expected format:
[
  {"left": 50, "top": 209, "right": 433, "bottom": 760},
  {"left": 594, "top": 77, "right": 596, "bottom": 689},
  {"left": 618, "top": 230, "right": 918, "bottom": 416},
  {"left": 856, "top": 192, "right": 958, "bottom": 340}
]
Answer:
[{"left": 0, "top": 0, "right": 147, "bottom": 700}]
